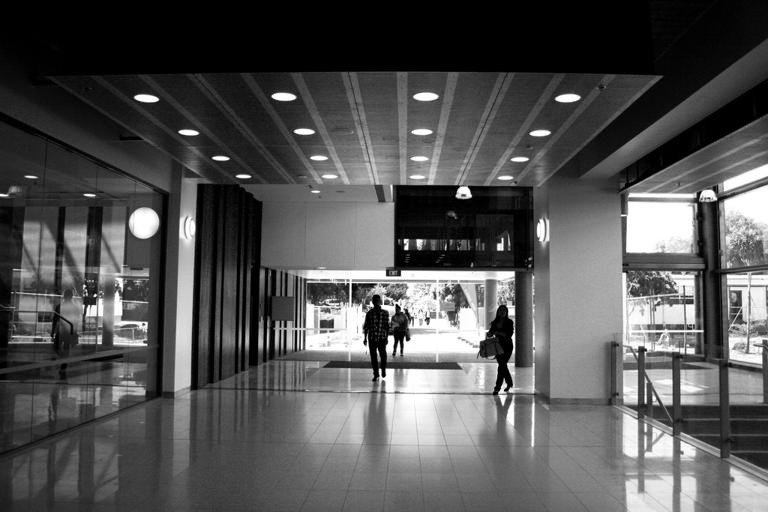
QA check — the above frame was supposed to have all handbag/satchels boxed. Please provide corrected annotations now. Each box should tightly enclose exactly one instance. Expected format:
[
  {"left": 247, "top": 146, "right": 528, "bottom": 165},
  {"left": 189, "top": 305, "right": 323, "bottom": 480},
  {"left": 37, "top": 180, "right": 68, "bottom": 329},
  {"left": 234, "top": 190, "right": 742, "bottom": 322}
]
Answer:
[
  {"left": 406, "top": 328, "right": 410, "bottom": 341},
  {"left": 480, "top": 337, "right": 504, "bottom": 357}
]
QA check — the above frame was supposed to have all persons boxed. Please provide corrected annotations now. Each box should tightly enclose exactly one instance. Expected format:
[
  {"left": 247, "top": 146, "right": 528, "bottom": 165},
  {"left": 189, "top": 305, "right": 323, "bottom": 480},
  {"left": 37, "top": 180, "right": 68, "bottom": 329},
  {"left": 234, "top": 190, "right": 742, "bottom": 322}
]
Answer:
[
  {"left": 487, "top": 304, "right": 514, "bottom": 394},
  {"left": 363, "top": 380, "right": 389, "bottom": 460},
  {"left": 51, "top": 289, "right": 82, "bottom": 382},
  {"left": 391, "top": 303, "right": 412, "bottom": 356},
  {"left": 404, "top": 308, "right": 412, "bottom": 323},
  {"left": 493, "top": 393, "right": 513, "bottom": 445},
  {"left": 363, "top": 294, "right": 390, "bottom": 382},
  {"left": 410, "top": 306, "right": 431, "bottom": 327}
]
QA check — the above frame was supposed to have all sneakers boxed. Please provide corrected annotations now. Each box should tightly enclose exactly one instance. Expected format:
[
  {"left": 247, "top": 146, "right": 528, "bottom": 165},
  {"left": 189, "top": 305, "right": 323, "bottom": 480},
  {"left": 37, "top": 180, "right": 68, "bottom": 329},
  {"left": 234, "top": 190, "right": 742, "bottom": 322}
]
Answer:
[
  {"left": 393, "top": 351, "right": 404, "bottom": 356},
  {"left": 493, "top": 384, "right": 513, "bottom": 396},
  {"left": 373, "top": 369, "right": 385, "bottom": 381}
]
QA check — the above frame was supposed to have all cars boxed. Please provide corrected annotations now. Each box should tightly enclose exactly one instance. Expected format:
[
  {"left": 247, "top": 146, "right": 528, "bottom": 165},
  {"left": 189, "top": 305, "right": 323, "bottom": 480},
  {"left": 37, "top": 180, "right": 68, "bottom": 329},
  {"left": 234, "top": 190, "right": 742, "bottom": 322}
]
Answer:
[{"left": 381, "top": 305, "right": 395, "bottom": 335}]
